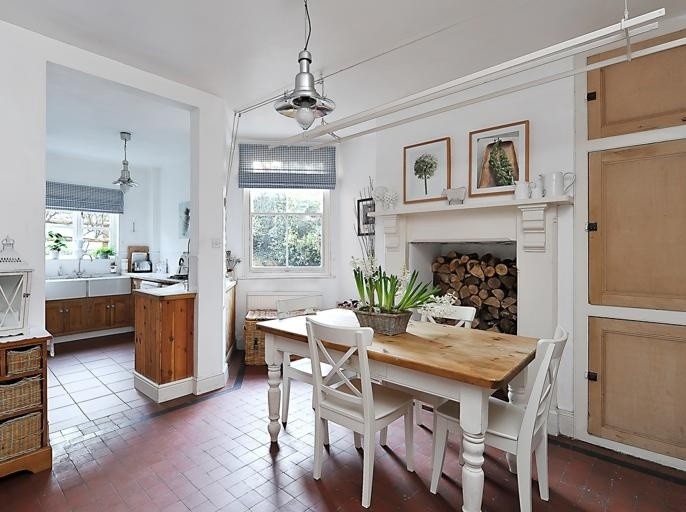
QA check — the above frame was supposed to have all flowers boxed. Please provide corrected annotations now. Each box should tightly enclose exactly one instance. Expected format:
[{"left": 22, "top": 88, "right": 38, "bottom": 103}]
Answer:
[{"left": 341, "top": 244, "right": 461, "bottom": 326}]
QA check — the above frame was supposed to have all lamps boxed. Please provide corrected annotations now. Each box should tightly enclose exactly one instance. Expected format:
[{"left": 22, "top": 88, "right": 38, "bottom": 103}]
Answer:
[
  {"left": 109, "top": 130, "right": 140, "bottom": 195},
  {"left": 273, "top": 1, "right": 335, "bottom": 130}
]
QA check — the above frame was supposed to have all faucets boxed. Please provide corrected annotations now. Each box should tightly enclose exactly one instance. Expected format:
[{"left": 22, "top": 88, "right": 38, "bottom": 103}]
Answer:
[{"left": 78, "top": 254, "right": 92, "bottom": 273}]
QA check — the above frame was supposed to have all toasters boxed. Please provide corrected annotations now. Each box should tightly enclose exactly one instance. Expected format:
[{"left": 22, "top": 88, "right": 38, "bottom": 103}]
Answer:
[{"left": 132, "top": 259, "right": 152, "bottom": 272}]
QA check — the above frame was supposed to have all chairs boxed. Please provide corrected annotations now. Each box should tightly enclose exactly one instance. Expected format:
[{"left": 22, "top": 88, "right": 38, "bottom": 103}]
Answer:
[
  {"left": 377, "top": 296, "right": 477, "bottom": 473},
  {"left": 300, "top": 312, "right": 418, "bottom": 510},
  {"left": 425, "top": 320, "right": 575, "bottom": 512},
  {"left": 274, "top": 292, "right": 364, "bottom": 452}
]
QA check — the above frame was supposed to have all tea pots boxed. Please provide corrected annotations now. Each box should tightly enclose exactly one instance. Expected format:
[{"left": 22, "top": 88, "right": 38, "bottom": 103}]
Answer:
[
  {"left": 177, "top": 257, "right": 187, "bottom": 276},
  {"left": 513, "top": 170, "right": 576, "bottom": 199}
]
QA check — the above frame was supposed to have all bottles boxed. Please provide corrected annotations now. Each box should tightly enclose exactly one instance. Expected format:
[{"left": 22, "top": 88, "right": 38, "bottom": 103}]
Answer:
[
  {"left": 226, "top": 250, "right": 236, "bottom": 272},
  {"left": 111, "top": 261, "right": 117, "bottom": 275},
  {"left": 183, "top": 280, "right": 189, "bottom": 292}
]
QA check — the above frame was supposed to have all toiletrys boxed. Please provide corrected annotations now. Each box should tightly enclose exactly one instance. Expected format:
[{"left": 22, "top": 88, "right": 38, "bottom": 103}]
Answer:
[{"left": 108, "top": 256, "right": 118, "bottom": 274}]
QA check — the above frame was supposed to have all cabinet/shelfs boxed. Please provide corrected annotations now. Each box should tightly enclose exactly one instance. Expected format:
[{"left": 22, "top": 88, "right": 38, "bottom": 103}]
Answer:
[
  {"left": 87, "top": 293, "right": 134, "bottom": 333},
  {"left": 43, "top": 297, "right": 89, "bottom": 338},
  {"left": 0, "top": 321, "right": 58, "bottom": 479},
  {"left": 130, "top": 273, "right": 179, "bottom": 333}
]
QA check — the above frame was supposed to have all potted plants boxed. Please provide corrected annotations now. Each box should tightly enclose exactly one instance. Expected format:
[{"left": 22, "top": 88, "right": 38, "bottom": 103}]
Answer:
[
  {"left": 90, "top": 245, "right": 116, "bottom": 260},
  {"left": 46, "top": 230, "right": 69, "bottom": 261}
]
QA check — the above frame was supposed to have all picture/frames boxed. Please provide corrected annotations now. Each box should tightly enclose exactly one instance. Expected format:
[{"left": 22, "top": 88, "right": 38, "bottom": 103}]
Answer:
[
  {"left": 353, "top": 196, "right": 376, "bottom": 237},
  {"left": 400, "top": 134, "right": 454, "bottom": 205},
  {"left": 466, "top": 118, "right": 531, "bottom": 197}
]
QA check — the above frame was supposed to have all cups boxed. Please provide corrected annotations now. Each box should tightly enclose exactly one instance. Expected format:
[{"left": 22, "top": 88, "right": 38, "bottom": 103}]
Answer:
[{"left": 120, "top": 258, "right": 128, "bottom": 273}]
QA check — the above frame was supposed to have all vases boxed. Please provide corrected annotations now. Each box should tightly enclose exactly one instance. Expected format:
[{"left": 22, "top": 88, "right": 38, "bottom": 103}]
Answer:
[{"left": 348, "top": 300, "right": 419, "bottom": 343}]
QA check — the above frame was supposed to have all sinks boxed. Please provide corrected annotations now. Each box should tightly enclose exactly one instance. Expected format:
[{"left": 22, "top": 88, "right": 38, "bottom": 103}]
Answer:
[
  {"left": 87, "top": 274, "right": 131, "bottom": 298},
  {"left": 45, "top": 278, "right": 87, "bottom": 301}
]
